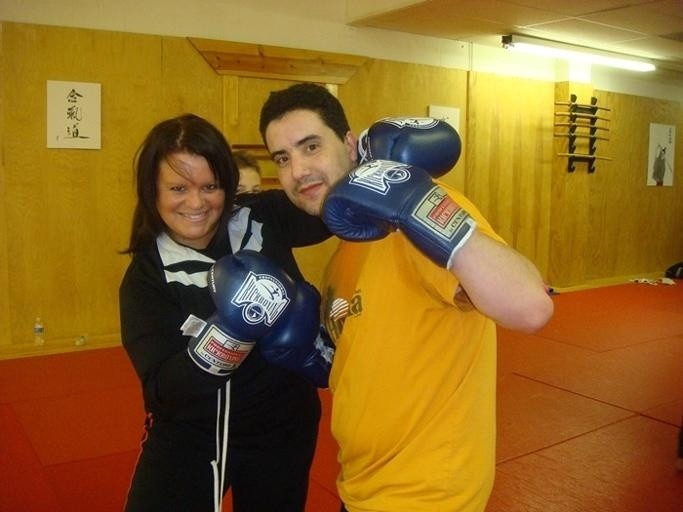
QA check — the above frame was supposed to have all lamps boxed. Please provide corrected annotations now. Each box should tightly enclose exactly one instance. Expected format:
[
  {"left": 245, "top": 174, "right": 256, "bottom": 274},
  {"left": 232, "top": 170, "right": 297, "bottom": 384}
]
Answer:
[{"left": 501, "top": 34, "right": 656, "bottom": 72}]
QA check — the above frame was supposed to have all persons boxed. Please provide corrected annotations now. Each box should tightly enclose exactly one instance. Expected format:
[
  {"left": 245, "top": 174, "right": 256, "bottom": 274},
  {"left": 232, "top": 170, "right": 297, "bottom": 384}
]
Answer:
[
  {"left": 118, "top": 115, "right": 461, "bottom": 511},
  {"left": 255, "top": 82, "right": 554, "bottom": 512}
]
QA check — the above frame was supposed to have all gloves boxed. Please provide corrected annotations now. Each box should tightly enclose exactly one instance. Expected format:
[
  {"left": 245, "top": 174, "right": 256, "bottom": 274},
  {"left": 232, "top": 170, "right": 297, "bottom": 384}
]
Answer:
[
  {"left": 181, "top": 249, "right": 296, "bottom": 376},
  {"left": 261, "top": 283, "right": 336, "bottom": 389},
  {"left": 322, "top": 160, "right": 476, "bottom": 270},
  {"left": 357, "top": 117, "right": 462, "bottom": 179}
]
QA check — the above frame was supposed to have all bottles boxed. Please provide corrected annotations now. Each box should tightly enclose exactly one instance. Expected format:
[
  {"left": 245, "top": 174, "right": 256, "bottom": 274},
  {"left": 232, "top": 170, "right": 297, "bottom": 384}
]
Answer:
[{"left": 33, "top": 317, "right": 43, "bottom": 346}]
